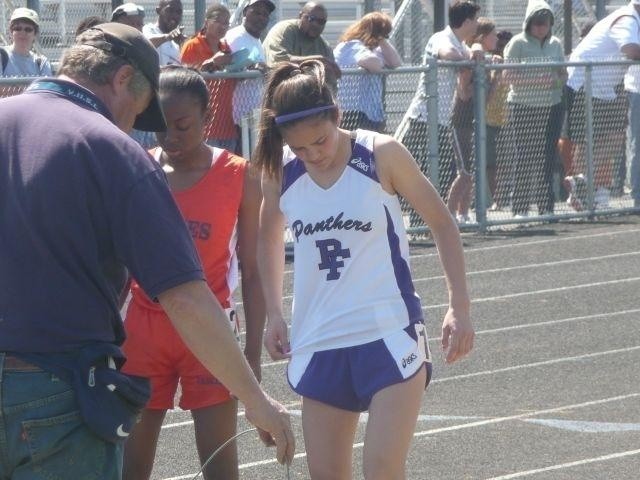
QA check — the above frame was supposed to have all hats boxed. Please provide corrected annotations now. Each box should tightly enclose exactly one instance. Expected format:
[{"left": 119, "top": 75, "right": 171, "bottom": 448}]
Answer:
[
  {"left": 111, "top": 2, "right": 145, "bottom": 22},
  {"left": 77, "top": 22, "right": 168, "bottom": 132},
  {"left": 243, "top": 0, "right": 275, "bottom": 17},
  {"left": 10, "top": 7, "right": 39, "bottom": 27}
]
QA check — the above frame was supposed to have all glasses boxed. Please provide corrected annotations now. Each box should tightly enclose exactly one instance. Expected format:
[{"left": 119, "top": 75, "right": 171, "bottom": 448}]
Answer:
[
  {"left": 302, "top": 13, "right": 326, "bottom": 25},
  {"left": 12, "top": 26, "right": 34, "bottom": 33}
]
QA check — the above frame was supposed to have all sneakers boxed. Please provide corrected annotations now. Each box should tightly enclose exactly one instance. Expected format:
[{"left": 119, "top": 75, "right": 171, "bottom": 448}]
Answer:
[{"left": 565, "top": 174, "right": 613, "bottom": 213}]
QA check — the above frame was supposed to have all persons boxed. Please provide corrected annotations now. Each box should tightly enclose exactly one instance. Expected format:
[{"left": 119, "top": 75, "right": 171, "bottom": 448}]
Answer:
[
  {"left": 113, "top": 62, "right": 266, "bottom": 480},
  {"left": 394, "top": 0, "right": 640, "bottom": 243},
  {"left": 1, "top": 19, "right": 298, "bottom": 480},
  {"left": 1, "top": 0, "right": 403, "bottom": 160},
  {"left": 252, "top": 56, "right": 477, "bottom": 479}
]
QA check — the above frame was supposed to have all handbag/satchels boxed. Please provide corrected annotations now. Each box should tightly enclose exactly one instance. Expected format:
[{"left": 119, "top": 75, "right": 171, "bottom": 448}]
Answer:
[{"left": 70, "top": 362, "right": 150, "bottom": 443}]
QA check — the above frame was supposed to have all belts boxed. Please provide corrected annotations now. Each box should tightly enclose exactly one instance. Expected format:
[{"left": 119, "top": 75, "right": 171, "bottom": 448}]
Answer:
[{"left": 4, "top": 354, "right": 40, "bottom": 371}]
